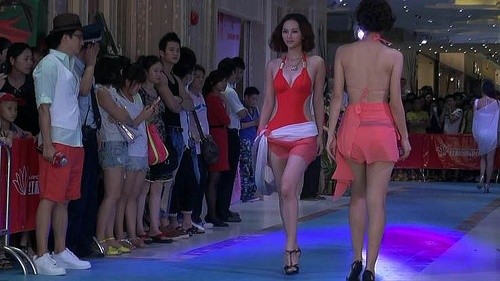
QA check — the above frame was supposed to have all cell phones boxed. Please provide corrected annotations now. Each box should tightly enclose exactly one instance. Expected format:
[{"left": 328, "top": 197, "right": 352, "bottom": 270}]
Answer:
[{"left": 146, "top": 96, "right": 161, "bottom": 110}]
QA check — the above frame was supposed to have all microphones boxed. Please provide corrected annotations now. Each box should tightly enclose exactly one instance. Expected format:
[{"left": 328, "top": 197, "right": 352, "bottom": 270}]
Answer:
[{"left": 33, "top": 146, "right": 68, "bottom": 168}]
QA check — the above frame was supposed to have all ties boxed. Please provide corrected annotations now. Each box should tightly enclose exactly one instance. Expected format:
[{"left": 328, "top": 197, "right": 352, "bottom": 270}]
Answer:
[{"left": 84, "top": 64, "right": 101, "bottom": 130}]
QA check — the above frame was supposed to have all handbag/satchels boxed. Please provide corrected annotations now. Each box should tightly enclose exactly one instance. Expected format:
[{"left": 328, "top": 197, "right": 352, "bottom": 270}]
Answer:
[
  {"left": 200, "top": 135, "right": 220, "bottom": 165},
  {"left": 117, "top": 121, "right": 140, "bottom": 144},
  {"left": 146, "top": 123, "right": 169, "bottom": 166}
]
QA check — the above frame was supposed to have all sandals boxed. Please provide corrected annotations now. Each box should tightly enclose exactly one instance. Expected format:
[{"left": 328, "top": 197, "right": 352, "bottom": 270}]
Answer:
[
  {"left": 15, "top": 246, "right": 35, "bottom": 257},
  {"left": 117, "top": 220, "right": 214, "bottom": 250},
  {"left": 0, "top": 252, "right": 13, "bottom": 268}
]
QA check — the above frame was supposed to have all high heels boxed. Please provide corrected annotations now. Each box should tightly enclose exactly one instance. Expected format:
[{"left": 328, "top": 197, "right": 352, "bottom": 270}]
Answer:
[
  {"left": 284, "top": 248, "right": 301, "bottom": 275},
  {"left": 346, "top": 260, "right": 362, "bottom": 281},
  {"left": 362, "top": 270, "right": 375, "bottom": 281},
  {"left": 484, "top": 184, "right": 490, "bottom": 193},
  {"left": 477, "top": 175, "right": 484, "bottom": 189}
]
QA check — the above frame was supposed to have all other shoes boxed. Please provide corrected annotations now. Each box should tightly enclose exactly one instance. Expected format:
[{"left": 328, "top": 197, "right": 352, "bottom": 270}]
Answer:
[
  {"left": 222, "top": 212, "right": 242, "bottom": 222},
  {"left": 306, "top": 195, "right": 326, "bottom": 201},
  {"left": 93, "top": 240, "right": 123, "bottom": 255},
  {"left": 246, "top": 197, "right": 261, "bottom": 203},
  {"left": 204, "top": 215, "right": 229, "bottom": 227},
  {"left": 105, "top": 238, "right": 129, "bottom": 253}
]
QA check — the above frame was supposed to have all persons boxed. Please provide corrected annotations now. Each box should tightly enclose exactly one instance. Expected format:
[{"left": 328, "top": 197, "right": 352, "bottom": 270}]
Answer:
[
  {"left": 318, "top": 64, "right": 356, "bottom": 196},
  {"left": 472, "top": 80, "right": 500, "bottom": 193},
  {"left": 327, "top": 0, "right": 412, "bottom": 281},
  {"left": 256, "top": 13, "right": 327, "bottom": 275},
  {"left": 398, "top": 77, "right": 478, "bottom": 182},
  {"left": 31, "top": 13, "right": 91, "bottom": 276},
  {"left": 0, "top": 23, "right": 263, "bottom": 257}
]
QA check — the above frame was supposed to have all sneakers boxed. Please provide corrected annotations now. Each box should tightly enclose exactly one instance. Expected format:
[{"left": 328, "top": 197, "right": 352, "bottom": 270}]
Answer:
[
  {"left": 33, "top": 253, "right": 66, "bottom": 276},
  {"left": 50, "top": 248, "right": 91, "bottom": 269}
]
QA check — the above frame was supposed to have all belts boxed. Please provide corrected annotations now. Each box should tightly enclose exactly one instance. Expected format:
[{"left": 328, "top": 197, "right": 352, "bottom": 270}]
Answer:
[
  {"left": 229, "top": 129, "right": 238, "bottom": 132},
  {"left": 209, "top": 125, "right": 224, "bottom": 128},
  {"left": 89, "top": 129, "right": 97, "bottom": 133},
  {"left": 170, "top": 127, "right": 183, "bottom": 133}
]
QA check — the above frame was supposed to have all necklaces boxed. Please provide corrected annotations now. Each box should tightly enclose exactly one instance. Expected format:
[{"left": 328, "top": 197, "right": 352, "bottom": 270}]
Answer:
[
  {"left": 118, "top": 88, "right": 136, "bottom": 104},
  {"left": 286, "top": 54, "right": 303, "bottom": 70}
]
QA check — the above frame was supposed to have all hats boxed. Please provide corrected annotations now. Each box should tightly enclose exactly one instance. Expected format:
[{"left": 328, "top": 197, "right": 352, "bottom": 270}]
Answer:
[
  {"left": 218, "top": 58, "right": 239, "bottom": 75},
  {"left": 82, "top": 24, "right": 102, "bottom": 43},
  {"left": 53, "top": 14, "right": 82, "bottom": 31},
  {"left": 0, "top": 92, "right": 25, "bottom": 105}
]
